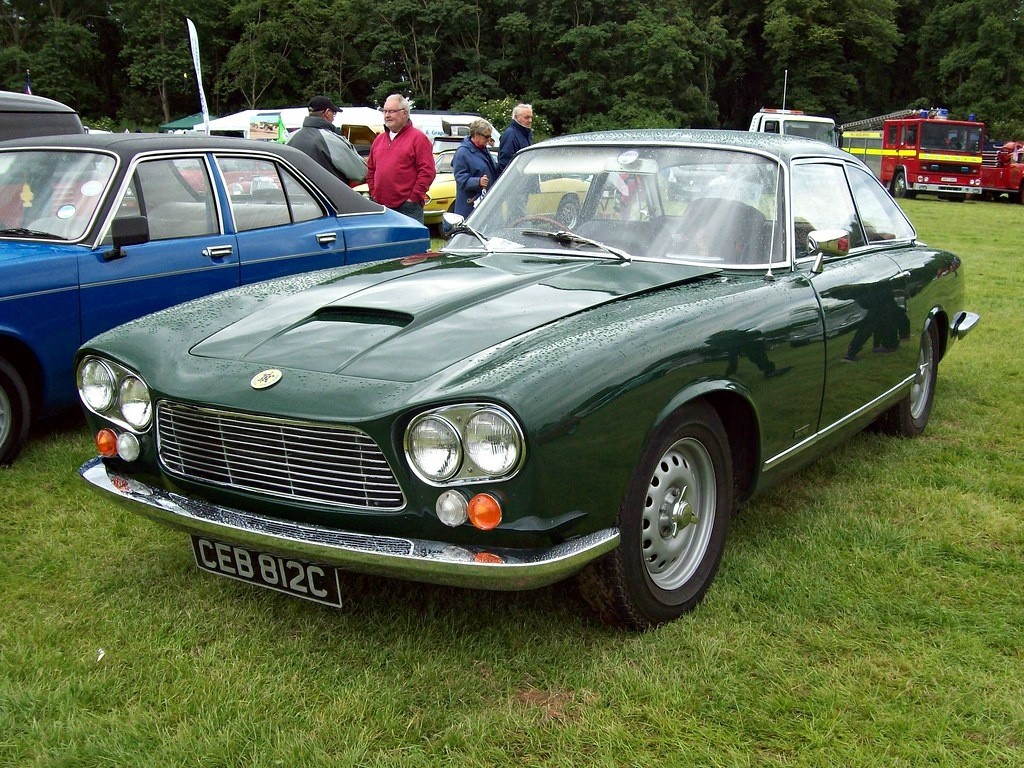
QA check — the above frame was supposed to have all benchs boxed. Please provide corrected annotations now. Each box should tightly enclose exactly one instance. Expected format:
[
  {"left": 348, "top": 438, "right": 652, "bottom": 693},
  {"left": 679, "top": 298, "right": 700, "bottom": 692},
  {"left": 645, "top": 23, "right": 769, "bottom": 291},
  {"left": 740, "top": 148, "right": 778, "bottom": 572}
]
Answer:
[
  {"left": 575, "top": 197, "right": 876, "bottom": 268},
  {"left": 146, "top": 200, "right": 321, "bottom": 238}
]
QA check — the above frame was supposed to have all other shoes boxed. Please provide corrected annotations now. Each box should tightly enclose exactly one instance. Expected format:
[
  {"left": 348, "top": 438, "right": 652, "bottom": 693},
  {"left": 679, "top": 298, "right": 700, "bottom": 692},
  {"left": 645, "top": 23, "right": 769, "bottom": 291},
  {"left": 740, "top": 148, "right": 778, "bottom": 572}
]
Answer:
[
  {"left": 841, "top": 355, "right": 858, "bottom": 364},
  {"left": 765, "top": 370, "right": 779, "bottom": 378}
]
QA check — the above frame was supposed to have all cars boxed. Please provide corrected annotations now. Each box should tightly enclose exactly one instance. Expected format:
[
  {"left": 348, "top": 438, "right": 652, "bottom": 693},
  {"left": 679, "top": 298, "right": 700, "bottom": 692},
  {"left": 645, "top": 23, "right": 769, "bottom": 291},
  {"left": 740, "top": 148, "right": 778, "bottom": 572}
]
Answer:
[
  {"left": 70, "top": 126, "right": 983, "bottom": 635},
  {"left": 0, "top": 130, "right": 432, "bottom": 470},
  {"left": 665, "top": 164, "right": 764, "bottom": 203},
  {"left": 349, "top": 148, "right": 621, "bottom": 241},
  {"left": 174, "top": 157, "right": 284, "bottom": 194}
]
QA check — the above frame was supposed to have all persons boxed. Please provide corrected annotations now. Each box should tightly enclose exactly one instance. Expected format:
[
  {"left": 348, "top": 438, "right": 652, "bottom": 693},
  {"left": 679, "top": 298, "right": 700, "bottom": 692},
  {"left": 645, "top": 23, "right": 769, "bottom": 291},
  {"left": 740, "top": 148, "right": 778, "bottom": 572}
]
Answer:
[
  {"left": 287, "top": 94, "right": 436, "bottom": 225},
  {"left": 453, "top": 104, "right": 534, "bottom": 221}
]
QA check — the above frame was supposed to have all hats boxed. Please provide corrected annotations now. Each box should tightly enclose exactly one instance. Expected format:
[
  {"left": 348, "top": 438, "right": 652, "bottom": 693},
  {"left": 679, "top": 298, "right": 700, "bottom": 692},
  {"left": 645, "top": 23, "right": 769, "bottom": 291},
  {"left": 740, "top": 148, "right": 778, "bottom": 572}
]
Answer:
[{"left": 307, "top": 96, "right": 343, "bottom": 113}]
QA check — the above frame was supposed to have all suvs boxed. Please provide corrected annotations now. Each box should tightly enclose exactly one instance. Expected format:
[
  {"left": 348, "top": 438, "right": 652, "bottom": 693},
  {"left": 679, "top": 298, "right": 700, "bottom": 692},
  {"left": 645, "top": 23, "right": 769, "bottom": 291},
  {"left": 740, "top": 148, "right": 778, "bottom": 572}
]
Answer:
[{"left": 0, "top": 90, "right": 100, "bottom": 183}]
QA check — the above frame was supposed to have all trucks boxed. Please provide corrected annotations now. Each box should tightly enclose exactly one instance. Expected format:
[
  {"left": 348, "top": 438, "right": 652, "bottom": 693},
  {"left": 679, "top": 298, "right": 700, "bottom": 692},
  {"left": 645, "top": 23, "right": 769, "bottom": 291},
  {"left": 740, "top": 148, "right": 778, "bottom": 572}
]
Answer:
[{"left": 748, "top": 108, "right": 845, "bottom": 194}]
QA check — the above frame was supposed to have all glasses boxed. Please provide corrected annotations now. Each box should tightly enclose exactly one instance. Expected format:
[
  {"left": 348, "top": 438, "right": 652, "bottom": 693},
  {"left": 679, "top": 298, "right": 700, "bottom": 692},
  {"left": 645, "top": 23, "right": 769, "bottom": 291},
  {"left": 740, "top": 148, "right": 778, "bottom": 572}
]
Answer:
[
  {"left": 478, "top": 133, "right": 491, "bottom": 138},
  {"left": 329, "top": 111, "right": 335, "bottom": 117},
  {"left": 382, "top": 108, "right": 404, "bottom": 115}
]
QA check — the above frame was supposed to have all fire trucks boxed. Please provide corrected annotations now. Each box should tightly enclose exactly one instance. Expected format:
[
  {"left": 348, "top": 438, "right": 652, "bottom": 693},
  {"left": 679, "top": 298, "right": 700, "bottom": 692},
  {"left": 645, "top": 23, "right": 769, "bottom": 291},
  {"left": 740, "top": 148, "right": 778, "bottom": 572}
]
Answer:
[{"left": 838, "top": 106, "right": 1024, "bottom": 206}]
